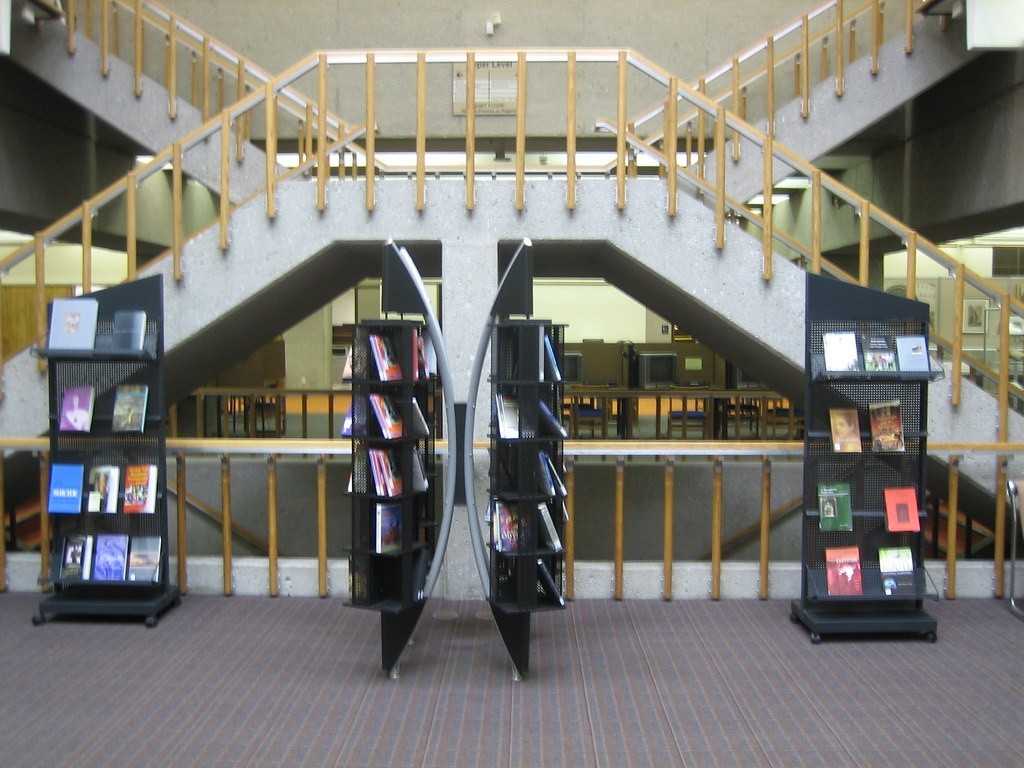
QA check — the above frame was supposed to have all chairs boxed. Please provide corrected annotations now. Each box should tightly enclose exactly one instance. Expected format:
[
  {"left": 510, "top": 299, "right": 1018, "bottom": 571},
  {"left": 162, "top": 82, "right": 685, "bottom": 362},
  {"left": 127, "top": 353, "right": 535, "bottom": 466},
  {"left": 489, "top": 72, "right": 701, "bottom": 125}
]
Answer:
[
  {"left": 242, "top": 382, "right": 287, "bottom": 438},
  {"left": 561, "top": 397, "right": 806, "bottom": 441}
]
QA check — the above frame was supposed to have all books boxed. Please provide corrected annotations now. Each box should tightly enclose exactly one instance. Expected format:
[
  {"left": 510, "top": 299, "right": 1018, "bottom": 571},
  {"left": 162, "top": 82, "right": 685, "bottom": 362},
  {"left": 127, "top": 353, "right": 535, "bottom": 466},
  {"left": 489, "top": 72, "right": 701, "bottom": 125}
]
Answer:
[
  {"left": 109, "top": 308, "right": 148, "bottom": 355},
  {"left": 59, "top": 385, "right": 148, "bottom": 434},
  {"left": 484, "top": 324, "right": 569, "bottom": 607},
  {"left": 47, "top": 462, "right": 158, "bottom": 514},
  {"left": 860, "top": 333, "right": 900, "bottom": 380},
  {"left": 342, "top": 327, "right": 430, "bottom": 601},
  {"left": 59, "top": 533, "right": 162, "bottom": 582},
  {"left": 822, "top": 330, "right": 862, "bottom": 382},
  {"left": 895, "top": 333, "right": 931, "bottom": 381},
  {"left": 817, "top": 398, "right": 920, "bottom": 601},
  {"left": 48, "top": 296, "right": 100, "bottom": 351}
]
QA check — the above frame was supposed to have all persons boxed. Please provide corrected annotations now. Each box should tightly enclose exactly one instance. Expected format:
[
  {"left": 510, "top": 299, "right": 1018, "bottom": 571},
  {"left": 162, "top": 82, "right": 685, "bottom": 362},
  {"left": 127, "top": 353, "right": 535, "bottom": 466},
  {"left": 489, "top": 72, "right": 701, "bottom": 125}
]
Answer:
[
  {"left": 97, "top": 473, "right": 109, "bottom": 513},
  {"left": 871, "top": 352, "right": 894, "bottom": 371},
  {"left": 833, "top": 412, "right": 862, "bottom": 452},
  {"left": 59, "top": 394, "right": 88, "bottom": 431}
]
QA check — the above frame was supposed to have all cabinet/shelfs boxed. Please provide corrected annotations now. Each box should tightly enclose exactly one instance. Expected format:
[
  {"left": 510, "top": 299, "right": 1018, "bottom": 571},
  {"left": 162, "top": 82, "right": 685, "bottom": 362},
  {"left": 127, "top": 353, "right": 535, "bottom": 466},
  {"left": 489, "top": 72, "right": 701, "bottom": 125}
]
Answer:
[
  {"left": 789, "top": 268, "right": 939, "bottom": 643},
  {"left": 31, "top": 275, "right": 181, "bottom": 624},
  {"left": 347, "top": 311, "right": 571, "bottom": 681}
]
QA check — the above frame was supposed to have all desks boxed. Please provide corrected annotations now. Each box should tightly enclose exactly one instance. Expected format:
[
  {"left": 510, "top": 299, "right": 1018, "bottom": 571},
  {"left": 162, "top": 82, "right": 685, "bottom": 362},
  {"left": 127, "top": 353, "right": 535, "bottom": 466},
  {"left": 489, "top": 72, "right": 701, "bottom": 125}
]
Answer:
[
  {"left": 167, "top": 386, "right": 352, "bottom": 440},
  {"left": 562, "top": 387, "right": 795, "bottom": 442}
]
